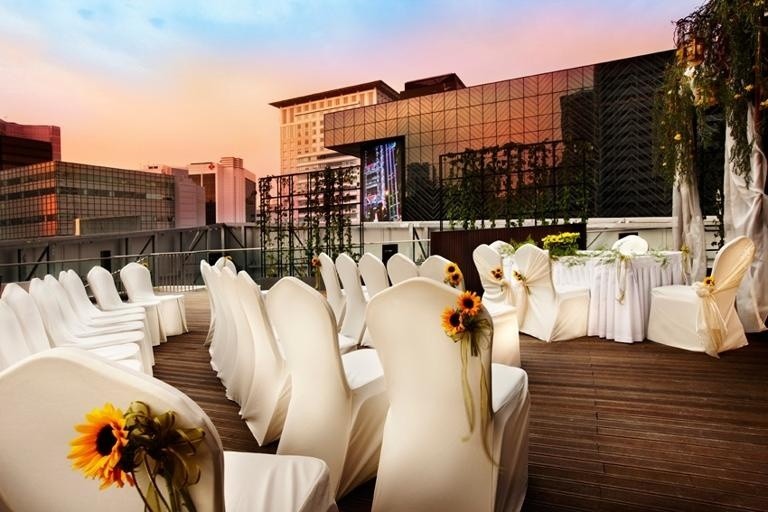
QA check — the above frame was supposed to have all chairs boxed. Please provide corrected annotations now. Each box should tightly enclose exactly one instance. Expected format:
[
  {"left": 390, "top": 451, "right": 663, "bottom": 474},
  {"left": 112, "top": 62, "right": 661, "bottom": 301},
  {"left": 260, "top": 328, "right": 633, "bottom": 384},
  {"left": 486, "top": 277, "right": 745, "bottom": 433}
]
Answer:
[
  {"left": 365, "top": 277, "right": 531, "bottom": 512},
  {"left": 201, "top": 253, "right": 520, "bottom": 499},
  {"left": 0, "top": 240, "right": 189, "bottom": 378},
  {"left": 472, "top": 233, "right": 756, "bottom": 360},
  {"left": 0, "top": 348, "right": 341, "bottom": 512}
]
